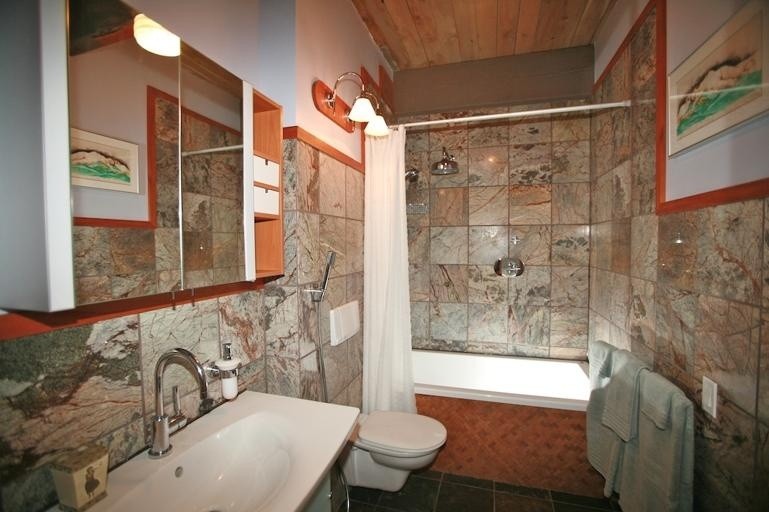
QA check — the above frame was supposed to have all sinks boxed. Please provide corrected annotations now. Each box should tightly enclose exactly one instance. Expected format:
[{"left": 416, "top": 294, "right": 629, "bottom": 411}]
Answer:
[{"left": 44, "top": 388, "right": 361, "bottom": 512}]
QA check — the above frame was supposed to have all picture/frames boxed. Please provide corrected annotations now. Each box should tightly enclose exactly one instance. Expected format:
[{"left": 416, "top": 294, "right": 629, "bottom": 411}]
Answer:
[
  {"left": 67, "top": 126, "right": 140, "bottom": 197},
  {"left": 663, "top": 0, "right": 769, "bottom": 161}
]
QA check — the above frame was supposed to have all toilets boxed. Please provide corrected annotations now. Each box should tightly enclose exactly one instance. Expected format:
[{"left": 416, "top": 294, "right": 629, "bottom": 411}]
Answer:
[{"left": 336, "top": 406, "right": 448, "bottom": 492}]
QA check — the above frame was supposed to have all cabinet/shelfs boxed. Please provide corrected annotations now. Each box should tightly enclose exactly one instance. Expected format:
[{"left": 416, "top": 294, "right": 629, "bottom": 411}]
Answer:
[
  {"left": 0, "top": 0, "right": 250, "bottom": 316},
  {"left": 249, "top": 83, "right": 287, "bottom": 283}
]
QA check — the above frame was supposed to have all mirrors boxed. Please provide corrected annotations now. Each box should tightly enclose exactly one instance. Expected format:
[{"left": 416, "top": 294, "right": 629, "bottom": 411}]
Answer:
[{"left": 56, "top": 0, "right": 251, "bottom": 306}]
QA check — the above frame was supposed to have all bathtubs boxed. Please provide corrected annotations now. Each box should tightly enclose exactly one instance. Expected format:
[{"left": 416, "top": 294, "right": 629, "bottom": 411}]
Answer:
[{"left": 409, "top": 347, "right": 605, "bottom": 500}]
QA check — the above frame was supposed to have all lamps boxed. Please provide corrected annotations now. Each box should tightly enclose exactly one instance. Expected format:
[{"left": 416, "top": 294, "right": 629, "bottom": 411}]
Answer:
[
  {"left": 126, "top": 5, "right": 184, "bottom": 63},
  {"left": 310, "top": 61, "right": 391, "bottom": 141}
]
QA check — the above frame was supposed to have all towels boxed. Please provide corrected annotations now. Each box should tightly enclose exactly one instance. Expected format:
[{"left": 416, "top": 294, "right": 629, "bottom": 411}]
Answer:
[{"left": 581, "top": 337, "right": 703, "bottom": 511}]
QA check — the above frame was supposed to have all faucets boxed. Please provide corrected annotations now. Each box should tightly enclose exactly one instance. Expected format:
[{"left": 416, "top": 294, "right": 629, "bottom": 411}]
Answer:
[{"left": 147, "top": 347, "right": 208, "bottom": 456}]
